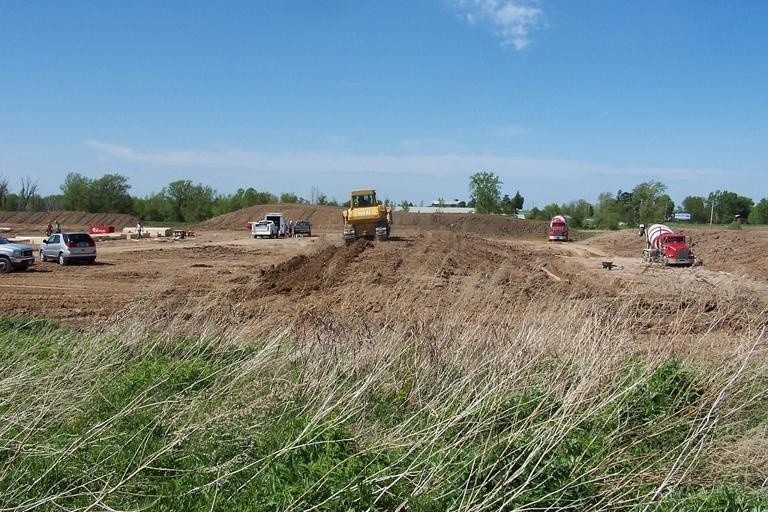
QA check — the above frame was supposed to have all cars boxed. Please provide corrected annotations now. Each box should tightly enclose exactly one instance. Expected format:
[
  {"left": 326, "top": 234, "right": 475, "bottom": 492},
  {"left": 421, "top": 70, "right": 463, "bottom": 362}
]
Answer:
[
  {"left": 0, "top": 235, "right": 35, "bottom": 274},
  {"left": 285, "top": 220, "right": 311, "bottom": 237}
]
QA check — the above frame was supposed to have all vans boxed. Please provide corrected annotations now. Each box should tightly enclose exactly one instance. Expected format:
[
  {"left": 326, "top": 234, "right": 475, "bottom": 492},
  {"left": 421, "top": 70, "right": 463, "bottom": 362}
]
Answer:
[{"left": 38, "top": 231, "right": 97, "bottom": 266}]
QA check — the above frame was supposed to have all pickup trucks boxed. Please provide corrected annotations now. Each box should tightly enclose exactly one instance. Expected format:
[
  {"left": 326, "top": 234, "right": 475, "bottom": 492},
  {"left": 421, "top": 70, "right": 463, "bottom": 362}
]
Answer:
[{"left": 251, "top": 219, "right": 277, "bottom": 239}]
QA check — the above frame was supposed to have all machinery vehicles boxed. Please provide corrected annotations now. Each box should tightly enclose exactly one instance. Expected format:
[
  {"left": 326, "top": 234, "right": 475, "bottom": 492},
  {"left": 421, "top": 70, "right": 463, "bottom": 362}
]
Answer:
[
  {"left": 548, "top": 214, "right": 569, "bottom": 241},
  {"left": 638, "top": 223, "right": 695, "bottom": 266},
  {"left": 341, "top": 189, "right": 393, "bottom": 245}
]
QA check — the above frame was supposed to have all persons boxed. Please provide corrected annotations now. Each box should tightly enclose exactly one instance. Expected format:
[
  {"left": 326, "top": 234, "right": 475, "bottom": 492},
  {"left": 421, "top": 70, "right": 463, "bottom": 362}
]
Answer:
[
  {"left": 46, "top": 220, "right": 61, "bottom": 237},
  {"left": 136, "top": 221, "right": 144, "bottom": 240}
]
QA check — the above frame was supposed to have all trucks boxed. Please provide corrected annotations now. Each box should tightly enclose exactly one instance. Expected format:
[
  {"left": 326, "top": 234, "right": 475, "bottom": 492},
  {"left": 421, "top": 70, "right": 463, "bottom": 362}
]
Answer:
[{"left": 264, "top": 212, "right": 287, "bottom": 235}]
